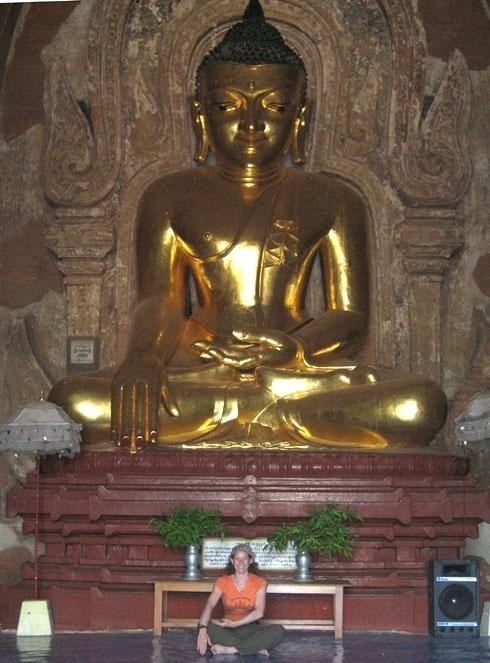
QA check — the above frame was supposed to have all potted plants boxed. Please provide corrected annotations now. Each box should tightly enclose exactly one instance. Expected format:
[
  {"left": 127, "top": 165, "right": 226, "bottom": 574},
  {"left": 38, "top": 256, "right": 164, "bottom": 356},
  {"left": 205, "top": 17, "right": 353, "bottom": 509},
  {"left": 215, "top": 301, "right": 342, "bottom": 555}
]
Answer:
[
  {"left": 263, "top": 503, "right": 364, "bottom": 582},
  {"left": 147, "top": 503, "right": 225, "bottom": 580}
]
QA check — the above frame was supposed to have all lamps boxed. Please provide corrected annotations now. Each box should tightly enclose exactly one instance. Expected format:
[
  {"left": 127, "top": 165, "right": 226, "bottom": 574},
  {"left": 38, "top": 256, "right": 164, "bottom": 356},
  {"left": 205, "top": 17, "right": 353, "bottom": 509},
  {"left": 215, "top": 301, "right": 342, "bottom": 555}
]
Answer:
[{"left": 0, "top": 386, "right": 84, "bottom": 635}]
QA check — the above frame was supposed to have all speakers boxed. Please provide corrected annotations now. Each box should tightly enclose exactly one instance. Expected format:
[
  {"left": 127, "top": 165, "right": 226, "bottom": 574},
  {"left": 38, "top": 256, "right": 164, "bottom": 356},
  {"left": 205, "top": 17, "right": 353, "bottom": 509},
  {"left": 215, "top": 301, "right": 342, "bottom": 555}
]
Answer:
[{"left": 430, "top": 559, "right": 480, "bottom": 638}]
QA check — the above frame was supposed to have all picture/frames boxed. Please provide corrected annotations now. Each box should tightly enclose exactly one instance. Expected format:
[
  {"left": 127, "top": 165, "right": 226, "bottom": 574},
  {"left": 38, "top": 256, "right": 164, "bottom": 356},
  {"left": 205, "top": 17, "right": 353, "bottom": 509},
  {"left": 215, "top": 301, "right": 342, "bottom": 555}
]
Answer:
[
  {"left": 66, "top": 336, "right": 99, "bottom": 370},
  {"left": 200, "top": 536, "right": 297, "bottom": 571}
]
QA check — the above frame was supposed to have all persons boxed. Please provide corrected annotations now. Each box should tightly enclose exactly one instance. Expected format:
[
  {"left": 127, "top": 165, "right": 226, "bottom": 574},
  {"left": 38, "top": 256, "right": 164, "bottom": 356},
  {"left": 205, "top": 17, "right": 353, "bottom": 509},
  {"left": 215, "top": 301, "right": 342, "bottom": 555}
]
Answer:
[
  {"left": 194, "top": 541, "right": 290, "bottom": 658},
  {"left": 48, "top": 0, "right": 455, "bottom": 456}
]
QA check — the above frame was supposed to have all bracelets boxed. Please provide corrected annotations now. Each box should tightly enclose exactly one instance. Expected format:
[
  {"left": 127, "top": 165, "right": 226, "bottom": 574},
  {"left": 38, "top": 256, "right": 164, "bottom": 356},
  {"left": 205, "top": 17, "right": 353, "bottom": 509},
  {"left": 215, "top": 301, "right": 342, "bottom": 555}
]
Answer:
[{"left": 198, "top": 623, "right": 207, "bottom": 630}]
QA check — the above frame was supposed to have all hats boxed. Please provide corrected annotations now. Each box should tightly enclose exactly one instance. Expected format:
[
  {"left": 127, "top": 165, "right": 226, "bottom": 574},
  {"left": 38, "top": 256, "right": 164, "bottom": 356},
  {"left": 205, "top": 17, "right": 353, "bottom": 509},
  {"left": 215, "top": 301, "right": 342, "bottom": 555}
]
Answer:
[{"left": 232, "top": 544, "right": 256, "bottom": 560}]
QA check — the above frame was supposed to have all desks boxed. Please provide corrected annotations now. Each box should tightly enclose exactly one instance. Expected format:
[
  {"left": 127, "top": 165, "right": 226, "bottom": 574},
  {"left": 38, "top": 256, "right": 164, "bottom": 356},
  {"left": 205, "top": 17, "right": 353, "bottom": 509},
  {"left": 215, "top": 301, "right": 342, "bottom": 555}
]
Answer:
[{"left": 146, "top": 576, "right": 352, "bottom": 638}]
